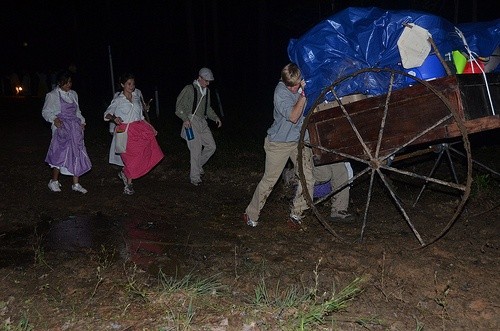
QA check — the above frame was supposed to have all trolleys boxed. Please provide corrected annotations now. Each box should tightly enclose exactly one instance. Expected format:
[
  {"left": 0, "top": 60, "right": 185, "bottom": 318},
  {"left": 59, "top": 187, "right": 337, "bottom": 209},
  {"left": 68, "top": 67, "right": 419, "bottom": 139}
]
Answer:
[{"left": 296, "top": 66, "right": 498, "bottom": 257}]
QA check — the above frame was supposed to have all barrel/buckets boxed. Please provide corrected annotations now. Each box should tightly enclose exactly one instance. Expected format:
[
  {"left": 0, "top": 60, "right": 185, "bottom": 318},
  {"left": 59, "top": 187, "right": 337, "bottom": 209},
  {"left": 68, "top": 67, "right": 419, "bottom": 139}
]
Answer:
[
  {"left": 406, "top": 54, "right": 446, "bottom": 86},
  {"left": 452, "top": 51, "right": 466, "bottom": 75},
  {"left": 463, "top": 59, "right": 484, "bottom": 74}
]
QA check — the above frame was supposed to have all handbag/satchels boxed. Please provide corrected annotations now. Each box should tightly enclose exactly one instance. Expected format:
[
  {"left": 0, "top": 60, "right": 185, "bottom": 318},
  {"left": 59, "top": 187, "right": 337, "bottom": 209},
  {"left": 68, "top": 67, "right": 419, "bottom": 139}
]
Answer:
[
  {"left": 181, "top": 121, "right": 188, "bottom": 140},
  {"left": 114, "top": 133, "right": 127, "bottom": 153}
]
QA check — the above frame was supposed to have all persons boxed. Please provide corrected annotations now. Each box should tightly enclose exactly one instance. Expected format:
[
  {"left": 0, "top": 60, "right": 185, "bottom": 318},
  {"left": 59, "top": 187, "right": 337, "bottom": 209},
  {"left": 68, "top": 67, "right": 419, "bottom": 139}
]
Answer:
[
  {"left": 175, "top": 68, "right": 223, "bottom": 188},
  {"left": 42, "top": 72, "right": 88, "bottom": 194},
  {"left": 104, "top": 75, "right": 145, "bottom": 196},
  {"left": 242, "top": 61, "right": 356, "bottom": 233}
]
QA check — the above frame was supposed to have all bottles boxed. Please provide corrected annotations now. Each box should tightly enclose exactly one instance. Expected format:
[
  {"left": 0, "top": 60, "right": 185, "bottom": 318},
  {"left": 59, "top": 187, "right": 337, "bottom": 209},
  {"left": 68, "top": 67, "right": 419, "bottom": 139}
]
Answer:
[{"left": 185, "top": 125, "right": 194, "bottom": 141}]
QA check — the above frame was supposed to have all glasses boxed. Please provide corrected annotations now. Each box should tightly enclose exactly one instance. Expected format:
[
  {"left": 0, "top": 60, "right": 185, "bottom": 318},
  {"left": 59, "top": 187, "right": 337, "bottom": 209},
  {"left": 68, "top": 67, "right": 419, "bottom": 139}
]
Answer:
[{"left": 199, "top": 75, "right": 208, "bottom": 82}]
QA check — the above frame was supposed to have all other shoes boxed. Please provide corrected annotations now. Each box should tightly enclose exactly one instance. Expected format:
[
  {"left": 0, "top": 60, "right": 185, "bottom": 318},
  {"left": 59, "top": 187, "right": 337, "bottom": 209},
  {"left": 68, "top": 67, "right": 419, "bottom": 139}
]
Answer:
[
  {"left": 118, "top": 171, "right": 127, "bottom": 186},
  {"left": 282, "top": 168, "right": 294, "bottom": 198},
  {"left": 330, "top": 210, "right": 352, "bottom": 219},
  {"left": 72, "top": 183, "right": 88, "bottom": 194},
  {"left": 200, "top": 172, "right": 205, "bottom": 177},
  {"left": 123, "top": 186, "right": 134, "bottom": 195},
  {"left": 287, "top": 218, "right": 307, "bottom": 232},
  {"left": 190, "top": 179, "right": 202, "bottom": 186},
  {"left": 240, "top": 213, "right": 258, "bottom": 228},
  {"left": 48, "top": 179, "right": 62, "bottom": 192}
]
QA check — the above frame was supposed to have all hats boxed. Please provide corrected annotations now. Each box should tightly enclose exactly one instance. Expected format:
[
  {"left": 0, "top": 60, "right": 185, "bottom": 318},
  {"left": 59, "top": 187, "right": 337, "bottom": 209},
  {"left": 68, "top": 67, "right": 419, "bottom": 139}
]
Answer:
[{"left": 199, "top": 67, "right": 214, "bottom": 80}]
